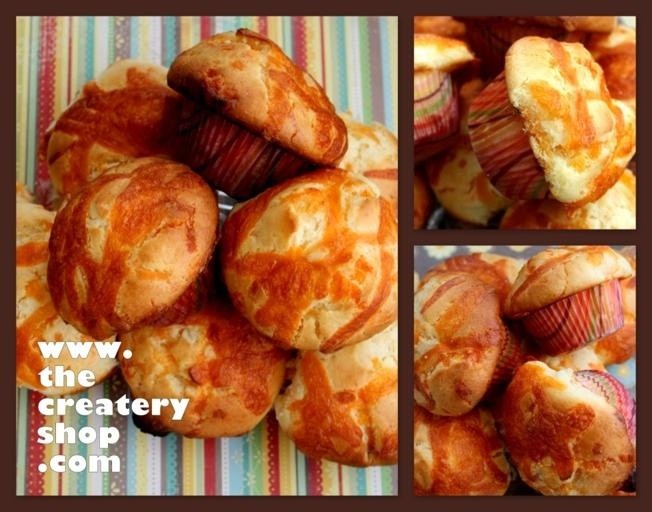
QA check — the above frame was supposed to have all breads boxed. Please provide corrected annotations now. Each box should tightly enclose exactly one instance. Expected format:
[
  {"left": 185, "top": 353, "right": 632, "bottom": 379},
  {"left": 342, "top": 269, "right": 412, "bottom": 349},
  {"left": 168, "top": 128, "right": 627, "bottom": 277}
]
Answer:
[
  {"left": 414, "top": 245, "right": 636, "bottom": 495},
  {"left": 16, "top": 28, "right": 398, "bottom": 468},
  {"left": 414, "top": 15, "right": 636, "bottom": 230}
]
